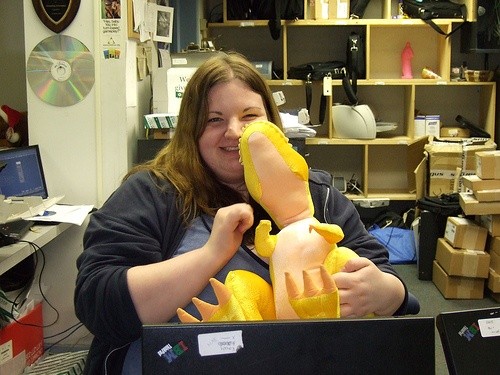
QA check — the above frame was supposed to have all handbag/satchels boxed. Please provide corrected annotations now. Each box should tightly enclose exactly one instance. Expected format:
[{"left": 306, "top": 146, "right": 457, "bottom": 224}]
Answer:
[
  {"left": 227, "top": 0, "right": 304, "bottom": 20},
  {"left": 367, "top": 212, "right": 417, "bottom": 264},
  {"left": 287, "top": 59, "right": 348, "bottom": 80},
  {"left": 477, "top": 0, "right": 500, "bottom": 49},
  {"left": 204, "top": 0, "right": 223, "bottom": 23},
  {"left": 346, "top": 32, "right": 366, "bottom": 79},
  {"left": 349, "top": 0, "right": 370, "bottom": 19},
  {"left": 401, "top": 0, "right": 467, "bottom": 19}
]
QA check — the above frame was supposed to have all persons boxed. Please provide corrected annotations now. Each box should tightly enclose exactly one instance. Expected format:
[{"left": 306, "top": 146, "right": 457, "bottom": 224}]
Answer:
[{"left": 74, "top": 50, "right": 408, "bottom": 375}]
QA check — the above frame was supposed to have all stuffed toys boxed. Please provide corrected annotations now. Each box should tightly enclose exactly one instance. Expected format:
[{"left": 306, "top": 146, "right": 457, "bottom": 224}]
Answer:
[
  {"left": 177, "top": 120, "right": 374, "bottom": 324},
  {"left": 0, "top": 104, "right": 23, "bottom": 147}
]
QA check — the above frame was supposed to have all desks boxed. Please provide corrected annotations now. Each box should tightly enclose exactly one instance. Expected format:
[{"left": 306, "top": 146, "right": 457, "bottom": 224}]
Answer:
[{"left": 0, "top": 212, "right": 94, "bottom": 344}]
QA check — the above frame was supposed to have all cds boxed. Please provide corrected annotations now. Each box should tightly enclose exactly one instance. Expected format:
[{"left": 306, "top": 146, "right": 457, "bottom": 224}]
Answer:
[{"left": 27, "top": 35, "right": 95, "bottom": 108}]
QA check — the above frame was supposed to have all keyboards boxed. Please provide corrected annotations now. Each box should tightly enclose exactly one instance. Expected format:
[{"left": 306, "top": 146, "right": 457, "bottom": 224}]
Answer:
[{"left": 0, "top": 219, "right": 34, "bottom": 247}]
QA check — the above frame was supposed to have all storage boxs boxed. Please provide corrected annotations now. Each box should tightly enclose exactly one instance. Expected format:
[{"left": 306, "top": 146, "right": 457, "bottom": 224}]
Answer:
[
  {"left": 413, "top": 116, "right": 500, "bottom": 299},
  {"left": 146, "top": 128, "right": 175, "bottom": 140},
  {"left": 152, "top": 67, "right": 196, "bottom": 114},
  {"left": 143, "top": 114, "right": 178, "bottom": 129},
  {"left": 0, "top": 303, "right": 44, "bottom": 375},
  {"left": 24, "top": 350, "right": 89, "bottom": 375},
  {"left": 309, "top": 0, "right": 349, "bottom": 20}
]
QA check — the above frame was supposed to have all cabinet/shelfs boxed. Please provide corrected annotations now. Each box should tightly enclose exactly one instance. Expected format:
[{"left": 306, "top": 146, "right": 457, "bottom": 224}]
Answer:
[{"left": 208, "top": 0, "right": 500, "bottom": 200}]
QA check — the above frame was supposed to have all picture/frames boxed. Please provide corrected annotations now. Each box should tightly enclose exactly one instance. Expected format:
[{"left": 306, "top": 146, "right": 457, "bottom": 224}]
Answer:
[{"left": 127, "top": 0, "right": 151, "bottom": 41}]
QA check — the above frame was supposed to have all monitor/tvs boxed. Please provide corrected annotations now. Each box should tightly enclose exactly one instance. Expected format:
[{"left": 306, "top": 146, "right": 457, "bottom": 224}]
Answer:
[
  {"left": 142, "top": 316, "right": 435, "bottom": 375},
  {"left": 435, "top": 308, "right": 500, "bottom": 375},
  {"left": 0, "top": 145, "right": 47, "bottom": 199}
]
